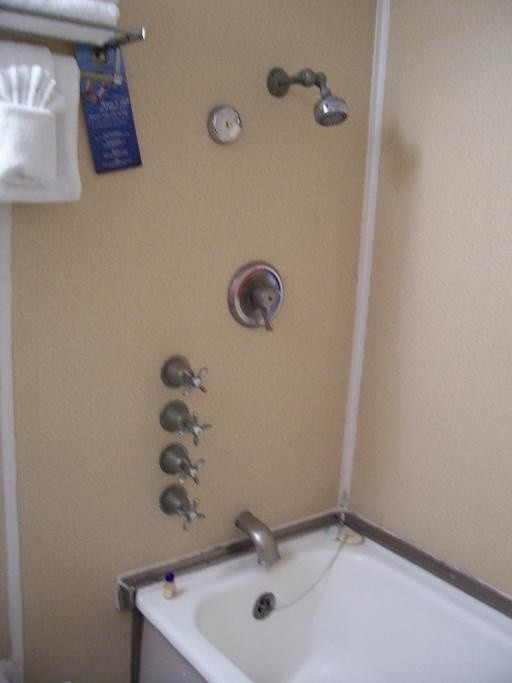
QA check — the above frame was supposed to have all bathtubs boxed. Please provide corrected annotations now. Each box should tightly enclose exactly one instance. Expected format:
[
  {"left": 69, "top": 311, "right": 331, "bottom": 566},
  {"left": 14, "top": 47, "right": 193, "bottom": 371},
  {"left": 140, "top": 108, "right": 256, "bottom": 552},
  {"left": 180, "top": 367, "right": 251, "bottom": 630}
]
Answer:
[{"left": 133, "top": 524, "right": 511, "bottom": 683}]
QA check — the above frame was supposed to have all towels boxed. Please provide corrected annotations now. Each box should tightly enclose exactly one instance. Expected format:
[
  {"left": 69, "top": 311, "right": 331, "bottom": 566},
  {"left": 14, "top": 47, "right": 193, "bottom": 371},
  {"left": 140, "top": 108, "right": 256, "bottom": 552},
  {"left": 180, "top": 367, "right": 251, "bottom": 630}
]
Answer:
[{"left": 1, "top": 0, "right": 119, "bottom": 204}]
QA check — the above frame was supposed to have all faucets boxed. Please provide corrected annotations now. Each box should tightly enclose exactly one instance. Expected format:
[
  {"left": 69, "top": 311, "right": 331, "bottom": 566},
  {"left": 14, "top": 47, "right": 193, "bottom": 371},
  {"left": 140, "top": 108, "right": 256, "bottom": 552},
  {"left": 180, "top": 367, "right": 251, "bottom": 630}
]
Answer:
[{"left": 235, "top": 509, "right": 282, "bottom": 566}]
